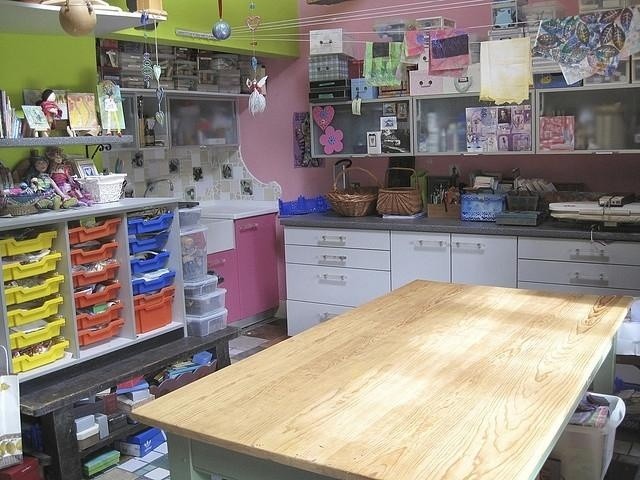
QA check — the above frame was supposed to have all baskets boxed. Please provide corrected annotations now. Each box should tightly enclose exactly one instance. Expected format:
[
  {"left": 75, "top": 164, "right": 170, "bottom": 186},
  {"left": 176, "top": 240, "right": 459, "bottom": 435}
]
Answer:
[
  {"left": 376, "top": 167, "right": 424, "bottom": 216},
  {"left": 326, "top": 167, "right": 382, "bottom": 217}
]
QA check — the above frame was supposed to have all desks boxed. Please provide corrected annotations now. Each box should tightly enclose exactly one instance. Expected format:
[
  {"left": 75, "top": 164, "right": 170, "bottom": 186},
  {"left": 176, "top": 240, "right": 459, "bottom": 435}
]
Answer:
[{"left": 130, "top": 278, "right": 636, "bottom": 479}]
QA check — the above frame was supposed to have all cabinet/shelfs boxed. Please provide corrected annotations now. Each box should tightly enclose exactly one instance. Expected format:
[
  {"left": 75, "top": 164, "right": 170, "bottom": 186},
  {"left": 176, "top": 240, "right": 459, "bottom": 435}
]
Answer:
[{"left": 272, "top": 0, "right": 640, "bottom": 363}]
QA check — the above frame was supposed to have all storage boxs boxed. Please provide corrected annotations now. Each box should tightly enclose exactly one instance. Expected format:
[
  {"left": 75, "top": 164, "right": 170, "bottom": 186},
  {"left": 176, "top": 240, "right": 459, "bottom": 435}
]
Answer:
[
  {"left": 530, "top": 382, "right": 631, "bottom": 480},
  {"left": 2, "top": 0, "right": 297, "bottom": 480}
]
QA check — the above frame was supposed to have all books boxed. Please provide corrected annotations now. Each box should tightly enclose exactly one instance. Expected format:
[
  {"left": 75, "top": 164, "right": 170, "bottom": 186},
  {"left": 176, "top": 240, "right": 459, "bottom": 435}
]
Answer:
[
  {"left": 382, "top": 211, "right": 427, "bottom": 220},
  {"left": 517, "top": 177, "right": 557, "bottom": 193}
]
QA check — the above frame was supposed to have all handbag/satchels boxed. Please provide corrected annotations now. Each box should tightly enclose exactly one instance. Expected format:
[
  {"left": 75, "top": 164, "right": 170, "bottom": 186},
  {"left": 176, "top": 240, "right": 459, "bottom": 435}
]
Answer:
[{"left": 0, "top": 345, "right": 24, "bottom": 470}]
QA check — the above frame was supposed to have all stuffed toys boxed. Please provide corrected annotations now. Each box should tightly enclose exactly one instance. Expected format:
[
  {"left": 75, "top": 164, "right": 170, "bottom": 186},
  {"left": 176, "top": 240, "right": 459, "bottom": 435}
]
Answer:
[
  {"left": 46, "top": 145, "right": 97, "bottom": 207},
  {"left": 21, "top": 155, "right": 77, "bottom": 212}
]
